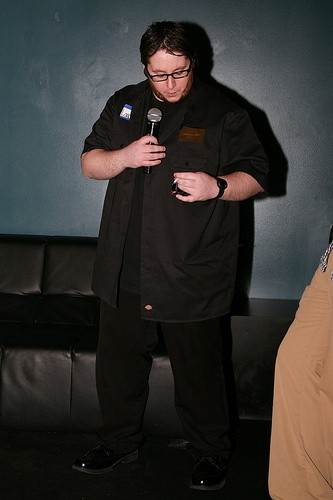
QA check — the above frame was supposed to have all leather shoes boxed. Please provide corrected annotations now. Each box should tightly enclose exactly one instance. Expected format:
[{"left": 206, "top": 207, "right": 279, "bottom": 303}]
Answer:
[
  {"left": 73, "top": 441, "right": 139, "bottom": 473},
  {"left": 189, "top": 453, "right": 228, "bottom": 489}
]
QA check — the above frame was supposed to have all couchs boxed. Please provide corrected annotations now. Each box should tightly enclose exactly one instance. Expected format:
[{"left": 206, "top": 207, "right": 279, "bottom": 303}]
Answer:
[{"left": 1, "top": 234, "right": 184, "bottom": 438}]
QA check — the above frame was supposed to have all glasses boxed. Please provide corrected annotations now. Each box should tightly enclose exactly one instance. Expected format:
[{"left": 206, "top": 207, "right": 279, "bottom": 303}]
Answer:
[{"left": 145, "top": 60, "right": 194, "bottom": 82}]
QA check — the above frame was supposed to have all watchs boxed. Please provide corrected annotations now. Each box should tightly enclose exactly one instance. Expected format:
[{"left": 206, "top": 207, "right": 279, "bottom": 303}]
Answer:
[{"left": 213, "top": 177, "right": 228, "bottom": 200}]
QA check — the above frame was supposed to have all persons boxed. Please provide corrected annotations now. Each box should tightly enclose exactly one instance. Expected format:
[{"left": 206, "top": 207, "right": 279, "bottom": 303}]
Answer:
[
  {"left": 267, "top": 239, "right": 333, "bottom": 500},
  {"left": 70, "top": 21, "right": 271, "bottom": 491}
]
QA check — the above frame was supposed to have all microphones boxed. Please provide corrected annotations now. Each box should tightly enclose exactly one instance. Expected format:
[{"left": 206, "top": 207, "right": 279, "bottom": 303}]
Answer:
[{"left": 145, "top": 107, "right": 162, "bottom": 173}]
